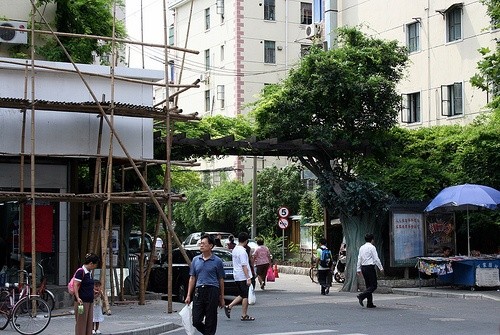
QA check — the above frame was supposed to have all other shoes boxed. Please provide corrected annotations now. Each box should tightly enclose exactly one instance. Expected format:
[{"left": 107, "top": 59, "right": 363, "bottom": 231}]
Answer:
[
  {"left": 367, "top": 304, "right": 376, "bottom": 308},
  {"left": 91, "top": 329, "right": 101, "bottom": 335},
  {"left": 260, "top": 281, "right": 266, "bottom": 289},
  {"left": 356, "top": 293, "right": 364, "bottom": 307}
]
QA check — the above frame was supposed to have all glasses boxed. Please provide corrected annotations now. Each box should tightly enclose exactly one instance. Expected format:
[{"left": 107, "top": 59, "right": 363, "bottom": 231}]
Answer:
[{"left": 199, "top": 242, "right": 210, "bottom": 245}]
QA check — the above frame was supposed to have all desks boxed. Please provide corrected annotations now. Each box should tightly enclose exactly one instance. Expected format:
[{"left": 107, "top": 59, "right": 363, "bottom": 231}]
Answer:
[{"left": 416, "top": 257, "right": 454, "bottom": 289}]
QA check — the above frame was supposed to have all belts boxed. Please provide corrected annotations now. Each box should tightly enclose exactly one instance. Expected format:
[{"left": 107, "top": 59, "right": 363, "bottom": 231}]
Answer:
[{"left": 200, "top": 285, "right": 211, "bottom": 288}]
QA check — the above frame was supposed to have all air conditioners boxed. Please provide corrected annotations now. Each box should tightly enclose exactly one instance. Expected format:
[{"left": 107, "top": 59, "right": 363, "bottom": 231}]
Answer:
[{"left": 304, "top": 24, "right": 319, "bottom": 39}]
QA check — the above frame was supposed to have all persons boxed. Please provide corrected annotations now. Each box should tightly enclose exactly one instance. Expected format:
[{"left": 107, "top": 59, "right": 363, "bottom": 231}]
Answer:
[
  {"left": 74, "top": 253, "right": 100, "bottom": 335},
  {"left": 316, "top": 239, "right": 332, "bottom": 294},
  {"left": 214, "top": 234, "right": 223, "bottom": 247},
  {"left": 92, "top": 280, "right": 105, "bottom": 335},
  {"left": 223, "top": 232, "right": 255, "bottom": 321},
  {"left": 185, "top": 234, "right": 225, "bottom": 335},
  {"left": 254, "top": 239, "right": 271, "bottom": 289},
  {"left": 244, "top": 240, "right": 256, "bottom": 289},
  {"left": 227, "top": 236, "right": 236, "bottom": 249},
  {"left": 358, "top": 234, "right": 384, "bottom": 308},
  {"left": 440, "top": 247, "right": 454, "bottom": 257}
]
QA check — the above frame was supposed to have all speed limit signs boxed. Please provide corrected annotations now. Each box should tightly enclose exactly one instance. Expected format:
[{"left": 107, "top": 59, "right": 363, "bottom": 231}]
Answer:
[{"left": 277, "top": 206, "right": 290, "bottom": 218}]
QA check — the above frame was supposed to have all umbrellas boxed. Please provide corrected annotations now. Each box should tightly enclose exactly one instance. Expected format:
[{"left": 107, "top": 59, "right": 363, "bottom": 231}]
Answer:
[{"left": 423, "top": 184, "right": 500, "bottom": 257}]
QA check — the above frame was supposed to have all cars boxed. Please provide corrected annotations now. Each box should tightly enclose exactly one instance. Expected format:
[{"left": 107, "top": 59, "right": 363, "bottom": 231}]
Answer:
[{"left": 129, "top": 230, "right": 259, "bottom": 296}]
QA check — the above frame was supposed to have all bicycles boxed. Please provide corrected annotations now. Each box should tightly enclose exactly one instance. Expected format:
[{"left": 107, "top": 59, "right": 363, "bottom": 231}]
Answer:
[
  {"left": 0, "top": 269, "right": 56, "bottom": 335},
  {"left": 309, "top": 266, "right": 320, "bottom": 284}
]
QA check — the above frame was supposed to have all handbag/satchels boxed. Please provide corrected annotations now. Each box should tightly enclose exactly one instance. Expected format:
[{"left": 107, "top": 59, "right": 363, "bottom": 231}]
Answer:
[
  {"left": 179, "top": 301, "right": 204, "bottom": 335},
  {"left": 266, "top": 264, "right": 275, "bottom": 282},
  {"left": 248, "top": 283, "right": 256, "bottom": 305}
]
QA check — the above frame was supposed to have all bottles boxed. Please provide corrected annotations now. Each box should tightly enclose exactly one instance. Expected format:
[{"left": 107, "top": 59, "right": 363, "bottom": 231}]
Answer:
[{"left": 78, "top": 305, "right": 84, "bottom": 314}]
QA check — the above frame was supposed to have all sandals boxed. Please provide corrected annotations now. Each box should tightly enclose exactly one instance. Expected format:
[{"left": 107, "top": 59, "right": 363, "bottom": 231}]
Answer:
[
  {"left": 224, "top": 305, "right": 231, "bottom": 318},
  {"left": 240, "top": 314, "right": 255, "bottom": 321}
]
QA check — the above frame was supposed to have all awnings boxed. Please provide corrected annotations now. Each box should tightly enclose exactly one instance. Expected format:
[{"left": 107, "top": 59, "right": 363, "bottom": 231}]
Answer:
[{"left": 304, "top": 219, "right": 341, "bottom": 259}]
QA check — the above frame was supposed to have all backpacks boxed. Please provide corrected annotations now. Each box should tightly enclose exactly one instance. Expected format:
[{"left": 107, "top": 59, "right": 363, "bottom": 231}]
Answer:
[
  {"left": 318, "top": 246, "right": 332, "bottom": 268},
  {"left": 68, "top": 267, "right": 85, "bottom": 297}
]
